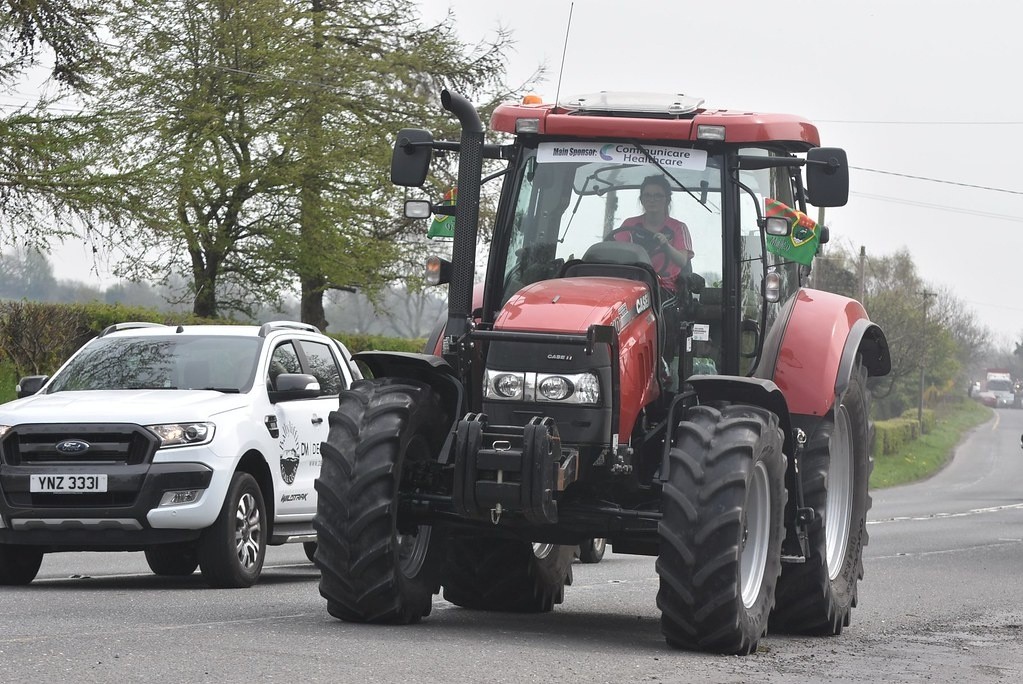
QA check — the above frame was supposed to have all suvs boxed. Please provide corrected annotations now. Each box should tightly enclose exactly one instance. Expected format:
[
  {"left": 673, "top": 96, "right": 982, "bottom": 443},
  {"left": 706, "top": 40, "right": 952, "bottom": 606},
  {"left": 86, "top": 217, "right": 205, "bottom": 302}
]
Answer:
[{"left": 0, "top": 320, "right": 365, "bottom": 590}]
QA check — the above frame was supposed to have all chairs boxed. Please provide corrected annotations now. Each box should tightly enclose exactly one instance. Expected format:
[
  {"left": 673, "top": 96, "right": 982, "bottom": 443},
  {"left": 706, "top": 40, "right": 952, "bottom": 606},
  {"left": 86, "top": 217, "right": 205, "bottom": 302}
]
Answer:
[{"left": 663, "top": 262, "right": 693, "bottom": 335}]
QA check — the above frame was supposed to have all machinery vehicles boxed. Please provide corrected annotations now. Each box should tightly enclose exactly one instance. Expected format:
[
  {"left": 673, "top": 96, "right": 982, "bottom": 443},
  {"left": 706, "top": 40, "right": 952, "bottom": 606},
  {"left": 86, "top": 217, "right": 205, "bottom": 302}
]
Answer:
[{"left": 312, "top": 87, "right": 893, "bottom": 656}]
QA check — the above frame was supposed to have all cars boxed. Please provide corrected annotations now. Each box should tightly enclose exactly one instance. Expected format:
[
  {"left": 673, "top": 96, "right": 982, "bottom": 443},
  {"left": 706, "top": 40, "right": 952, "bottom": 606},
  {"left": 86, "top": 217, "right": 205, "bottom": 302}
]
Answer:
[{"left": 969, "top": 381, "right": 996, "bottom": 407}]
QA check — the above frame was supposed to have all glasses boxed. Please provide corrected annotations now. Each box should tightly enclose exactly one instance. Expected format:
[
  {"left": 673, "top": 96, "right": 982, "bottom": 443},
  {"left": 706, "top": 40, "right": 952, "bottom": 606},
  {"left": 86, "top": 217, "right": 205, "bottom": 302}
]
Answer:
[{"left": 639, "top": 193, "right": 668, "bottom": 201}]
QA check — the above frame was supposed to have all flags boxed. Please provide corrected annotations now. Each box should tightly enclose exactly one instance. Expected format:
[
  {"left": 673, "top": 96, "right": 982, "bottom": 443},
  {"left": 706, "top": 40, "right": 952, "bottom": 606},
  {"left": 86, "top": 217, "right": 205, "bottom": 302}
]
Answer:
[
  {"left": 765, "top": 198, "right": 821, "bottom": 267},
  {"left": 427, "top": 186, "right": 458, "bottom": 239}
]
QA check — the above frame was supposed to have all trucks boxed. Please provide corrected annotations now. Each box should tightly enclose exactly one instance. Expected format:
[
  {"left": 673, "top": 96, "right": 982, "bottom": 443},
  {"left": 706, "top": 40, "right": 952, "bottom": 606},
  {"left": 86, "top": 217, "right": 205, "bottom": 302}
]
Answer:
[{"left": 981, "top": 368, "right": 1017, "bottom": 408}]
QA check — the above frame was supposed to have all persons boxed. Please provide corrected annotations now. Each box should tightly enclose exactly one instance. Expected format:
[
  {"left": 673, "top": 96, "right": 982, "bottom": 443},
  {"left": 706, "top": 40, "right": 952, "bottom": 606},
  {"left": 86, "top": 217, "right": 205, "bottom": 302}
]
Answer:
[
  {"left": 968, "top": 383, "right": 973, "bottom": 398},
  {"left": 615, "top": 174, "right": 692, "bottom": 378}
]
